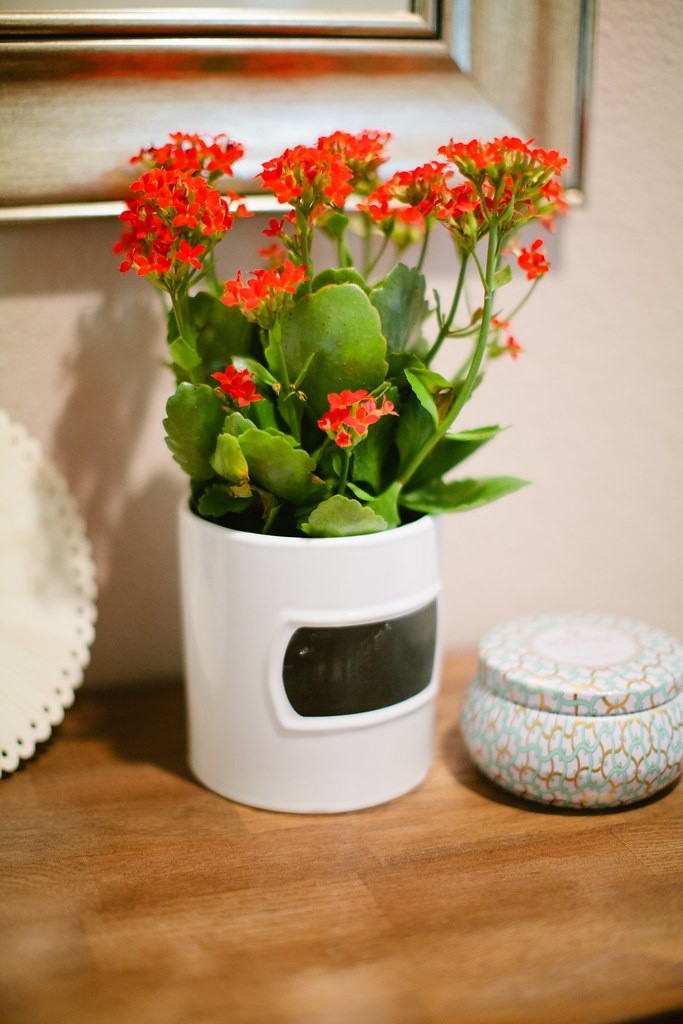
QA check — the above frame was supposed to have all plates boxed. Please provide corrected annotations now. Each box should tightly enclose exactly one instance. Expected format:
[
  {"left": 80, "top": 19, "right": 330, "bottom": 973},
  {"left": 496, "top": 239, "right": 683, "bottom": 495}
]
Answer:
[{"left": 0, "top": 408, "right": 100, "bottom": 780}]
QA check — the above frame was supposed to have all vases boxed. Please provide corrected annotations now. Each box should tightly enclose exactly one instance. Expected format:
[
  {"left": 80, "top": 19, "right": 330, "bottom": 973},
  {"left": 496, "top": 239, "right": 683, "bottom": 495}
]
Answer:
[{"left": 175, "top": 495, "right": 437, "bottom": 815}]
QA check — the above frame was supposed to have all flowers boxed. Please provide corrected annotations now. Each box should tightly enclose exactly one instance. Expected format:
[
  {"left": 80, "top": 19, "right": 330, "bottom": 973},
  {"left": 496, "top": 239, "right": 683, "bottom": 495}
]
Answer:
[{"left": 112, "top": 115, "right": 575, "bottom": 532}]
